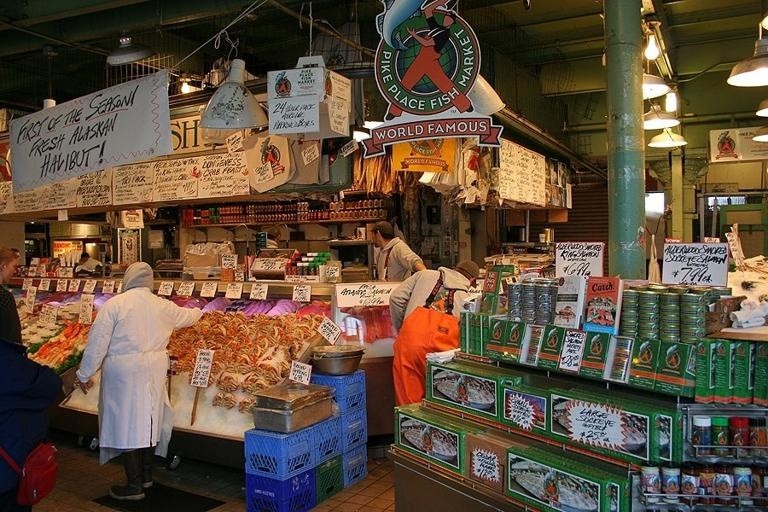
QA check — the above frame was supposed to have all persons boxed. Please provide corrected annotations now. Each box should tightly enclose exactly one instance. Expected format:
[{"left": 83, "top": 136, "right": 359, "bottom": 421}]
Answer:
[
  {"left": 0, "top": 337, "right": 63, "bottom": 512},
  {"left": 276, "top": 76, "right": 289, "bottom": 97},
  {"left": 76, "top": 262, "right": 203, "bottom": 501},
  {"left": 385, "top": 0, "right": 474, "bottom": 121},
  {"left": 264, "top": 145, "right": 280, "bottom": 165},
  {"left": 0, "top": 246, "right": 23, "bottom": 344},
  {"left": 370, "top": 221, "right": 427, "bottom": 281},
  {"left": 389, "top": 260, "right": 480, "bottom": 405}
]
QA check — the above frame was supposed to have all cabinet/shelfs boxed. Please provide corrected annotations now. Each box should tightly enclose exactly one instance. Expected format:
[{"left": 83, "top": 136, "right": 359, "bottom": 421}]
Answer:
[
  {"left": 8, "top": 278, "right": 404, "bottom": 473},
  {"left": 179, "top": 204, "right": 387, "bottom": 283},
  {"left": 501, "top": 242, "right": 556, "bottom": 255},
  {"left": 387, "top": 451, "right": 533, "bottom": 511}
]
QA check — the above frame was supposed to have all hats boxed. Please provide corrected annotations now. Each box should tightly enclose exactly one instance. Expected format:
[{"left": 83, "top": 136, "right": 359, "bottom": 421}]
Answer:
[
  {"left": 367, "top": 222, "right": 393, "bottom": 236},
  {"left": 456, "top": 260, "right": 479, "bottom": 288}
]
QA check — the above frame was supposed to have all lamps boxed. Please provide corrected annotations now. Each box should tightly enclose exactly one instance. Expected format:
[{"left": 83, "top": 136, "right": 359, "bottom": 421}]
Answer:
[
  {"left": 106, "top": 30, "right": 156, "bottom": 66},
  {"left": 642, "top": 13, "right": 662, "bottom": 60},
  {"left": 648, "top": 127, "right": 688, "bottom": 147},
  {"left": 727, "top": 22, "right": 768, "bottom": 87},
  {"left": 756, "top": 98, "right": 768, "bottom": 117},
  {"left": 198, "top": 58, "right": 269, "bottom": 129},
  {"left": 644, "top": 99, "right": 680, "bottom": 130},
  {"left": 643, "top": 68, "right": 672, "bottom": 99},
  {"left": 752, "top": 122, "right": 768, "bottom": 142}
]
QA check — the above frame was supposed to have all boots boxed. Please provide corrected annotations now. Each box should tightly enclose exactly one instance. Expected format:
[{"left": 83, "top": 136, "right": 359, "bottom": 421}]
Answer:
[{"left": 109, "top": 451, "right": 153, "bottom": 500}]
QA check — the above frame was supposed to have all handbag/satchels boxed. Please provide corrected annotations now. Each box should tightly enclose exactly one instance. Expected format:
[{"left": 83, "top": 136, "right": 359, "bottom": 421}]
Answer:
[{"left": 16, "top": 444, "right": 58, "bottom": 504}]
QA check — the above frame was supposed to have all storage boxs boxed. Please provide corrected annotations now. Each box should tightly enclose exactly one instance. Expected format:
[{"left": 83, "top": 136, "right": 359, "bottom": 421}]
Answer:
[{"left": 664, "top": 185, "right": 696, "bottom": 213}]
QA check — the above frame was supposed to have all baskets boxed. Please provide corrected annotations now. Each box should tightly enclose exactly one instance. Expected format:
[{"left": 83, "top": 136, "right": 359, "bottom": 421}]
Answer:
[
  {"left": 309, "top": 369, "right": 367, "bottom": 417},
  {"left": 244, "top": 409, "right": 369, "bottom": 511}
]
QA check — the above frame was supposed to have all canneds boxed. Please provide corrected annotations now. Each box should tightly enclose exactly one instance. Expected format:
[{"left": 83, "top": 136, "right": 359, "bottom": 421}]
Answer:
[
  {"left": 640, "top": 461, "right": 768, "bottom": 506},
  {"left": 620, "top": 280, "right": 732, "bottom": 345},
  {"left": 193, "top": 198, "right": 385, "bottom": 224},
  {"left": 692, "top": 414, "right": 768, "bottom": 458},
  {"left": 286, "top": 251, "right": 331, "bottom": 275},
  {"left": 508, "top": 277, "right": 559, "bottom": 324}
]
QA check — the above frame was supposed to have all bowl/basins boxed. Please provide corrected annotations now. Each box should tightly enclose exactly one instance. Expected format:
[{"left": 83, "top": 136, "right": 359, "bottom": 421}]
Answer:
[{"left": 310, "top": 344, "right": 368, "bottom": 375}]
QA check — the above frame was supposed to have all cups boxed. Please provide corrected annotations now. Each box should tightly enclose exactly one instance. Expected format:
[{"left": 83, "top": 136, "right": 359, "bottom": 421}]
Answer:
[
  {"left": 539, "top": 234, "right": 546, "bottom": 242},
  {"left": 357, "top": 228, "right": 365, "bottom": 240}
]
